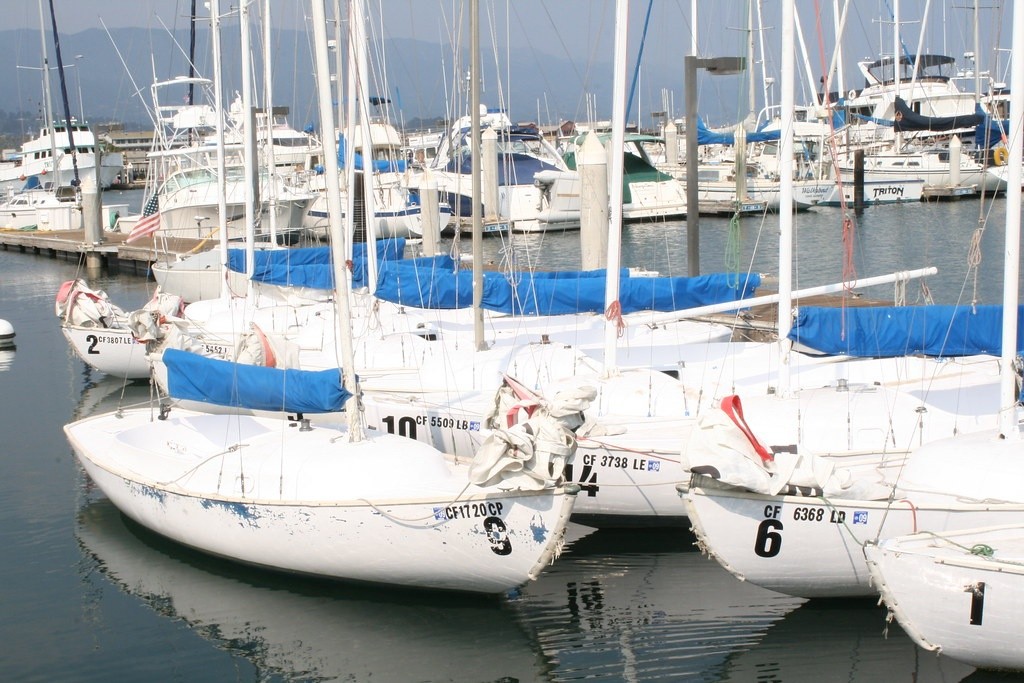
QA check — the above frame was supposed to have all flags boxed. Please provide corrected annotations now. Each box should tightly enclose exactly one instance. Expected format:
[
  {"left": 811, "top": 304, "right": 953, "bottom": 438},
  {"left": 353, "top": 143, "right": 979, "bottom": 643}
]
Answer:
[{"left": 126, "top": 193, "right": 160, "bottom": 245}]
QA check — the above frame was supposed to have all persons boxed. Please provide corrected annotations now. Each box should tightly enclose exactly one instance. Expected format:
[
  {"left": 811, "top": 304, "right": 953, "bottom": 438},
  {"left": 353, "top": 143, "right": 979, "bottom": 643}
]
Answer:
[
  {"left": 416, "top": 151, "right": 426, "bottom": 164},
  {"left": 911, "top": 136, "right": 936, "bottom": 149}
]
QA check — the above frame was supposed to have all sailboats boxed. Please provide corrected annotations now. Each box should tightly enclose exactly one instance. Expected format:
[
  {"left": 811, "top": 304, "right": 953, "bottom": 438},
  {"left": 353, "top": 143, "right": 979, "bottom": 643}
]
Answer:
[{"left": 1, "top": 1, "right": 1023, "bottom": 675}]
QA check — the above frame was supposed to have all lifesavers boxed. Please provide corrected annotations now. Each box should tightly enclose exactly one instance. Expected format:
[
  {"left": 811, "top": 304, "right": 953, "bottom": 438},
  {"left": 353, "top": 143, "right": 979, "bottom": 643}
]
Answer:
[
  {"left": 994, "top": 146, "right": 1009, "bottom": 165},
  {"left": 848, "top": 89, "right": 856, "bottom": 100}
]
